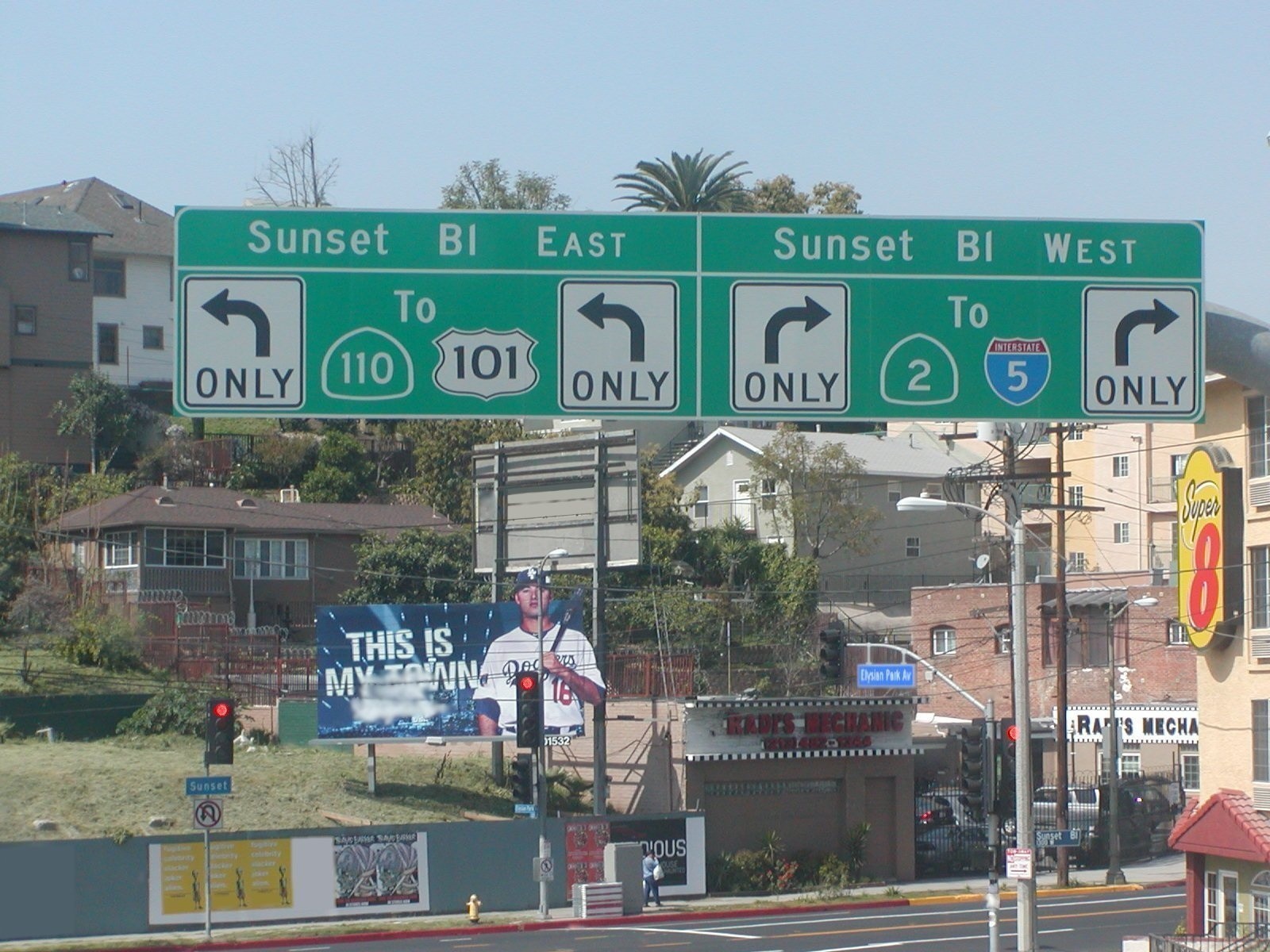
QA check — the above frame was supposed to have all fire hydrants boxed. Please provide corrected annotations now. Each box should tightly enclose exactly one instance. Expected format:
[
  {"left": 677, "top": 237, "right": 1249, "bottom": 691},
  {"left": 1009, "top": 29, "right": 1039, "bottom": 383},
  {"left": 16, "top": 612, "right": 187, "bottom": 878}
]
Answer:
[{"left": 465, "top": 894, "right": 483, "bottom": 924}]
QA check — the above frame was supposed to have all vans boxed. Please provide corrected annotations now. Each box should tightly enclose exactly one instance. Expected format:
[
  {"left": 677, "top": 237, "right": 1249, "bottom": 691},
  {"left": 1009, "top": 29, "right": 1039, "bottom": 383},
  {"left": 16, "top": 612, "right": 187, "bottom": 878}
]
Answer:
[{"left": 996, "top": 786, "right": 1100, "bottom": 836}]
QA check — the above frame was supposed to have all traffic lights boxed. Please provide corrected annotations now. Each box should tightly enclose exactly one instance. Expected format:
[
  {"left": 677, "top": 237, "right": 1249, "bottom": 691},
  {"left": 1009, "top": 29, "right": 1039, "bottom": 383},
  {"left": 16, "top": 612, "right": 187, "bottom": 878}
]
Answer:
[
  {"left": 818, "top": 628, "right": 847, "bottom": 685},
  {"left": 515, "top": 671, "right": 544, "bottom": 748},
  {"left": 511, "top": 752, "right": 532, "bottom": 801},
  {"left": 1001, "top": 717, "right": 1031, "bottom": 818},
  {"left": 957, "top": 718, "right": 986, "bottom": 824},
  {"left": 204, "top": 699, "right": 233, "bottom": 764}
]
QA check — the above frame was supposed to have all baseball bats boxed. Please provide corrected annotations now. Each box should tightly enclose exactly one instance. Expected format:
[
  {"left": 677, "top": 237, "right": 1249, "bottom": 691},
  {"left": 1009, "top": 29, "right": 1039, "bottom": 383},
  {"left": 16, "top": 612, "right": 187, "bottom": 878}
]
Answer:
[{"left": 543, "top": 586, "right": 589, "bottom": 671}]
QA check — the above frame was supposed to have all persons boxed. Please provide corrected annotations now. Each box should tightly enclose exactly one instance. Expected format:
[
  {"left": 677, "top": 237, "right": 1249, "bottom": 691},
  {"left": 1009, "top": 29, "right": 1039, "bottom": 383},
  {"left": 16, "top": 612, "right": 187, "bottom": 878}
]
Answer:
[
  {"left": 642, "top": 849, "right": 664, "bottom": 907},
  {"left": 472, "top": 568, "right": 607, "bottom": 737}
]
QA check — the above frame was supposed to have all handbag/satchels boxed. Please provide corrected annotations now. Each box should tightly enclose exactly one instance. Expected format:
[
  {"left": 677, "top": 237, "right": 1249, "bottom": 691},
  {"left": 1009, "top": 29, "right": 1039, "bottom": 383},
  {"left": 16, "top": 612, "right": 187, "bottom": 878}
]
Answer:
[{"left": 653, "top": 865, "right": 664, "bottom": 881}]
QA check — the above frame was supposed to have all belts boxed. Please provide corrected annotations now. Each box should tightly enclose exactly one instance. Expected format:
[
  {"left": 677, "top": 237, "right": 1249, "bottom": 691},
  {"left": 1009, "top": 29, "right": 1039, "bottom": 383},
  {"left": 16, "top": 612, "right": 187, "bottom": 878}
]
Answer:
[{"left": 507, "top": 726, "right": 576, "bottom": 733}]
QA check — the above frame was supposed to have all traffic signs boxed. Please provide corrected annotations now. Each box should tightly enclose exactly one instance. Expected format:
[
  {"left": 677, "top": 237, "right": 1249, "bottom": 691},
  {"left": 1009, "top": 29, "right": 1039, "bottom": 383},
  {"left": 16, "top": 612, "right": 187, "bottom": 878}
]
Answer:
[
  {"left": 1081, "top": 286, "right": 1207, "bottom": 424},
  {"left": 180, "top": 273, "right": 307, "bottom": 408},
  {"left": 558, "top": 279, "right": 683, "bottom": 413},
  {"left": 729, "top": 278, "right": 853, "bottom": 413}
]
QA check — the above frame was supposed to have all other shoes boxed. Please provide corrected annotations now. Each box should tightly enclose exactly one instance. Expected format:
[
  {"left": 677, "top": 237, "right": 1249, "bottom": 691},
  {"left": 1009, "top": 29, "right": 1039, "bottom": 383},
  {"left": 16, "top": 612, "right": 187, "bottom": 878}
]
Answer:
[
  {"left": 656, "top": 904, "right": 662, "bottom": 907},
  {"left": 643, "top": 904, "right": 650, "bottom": 907}
]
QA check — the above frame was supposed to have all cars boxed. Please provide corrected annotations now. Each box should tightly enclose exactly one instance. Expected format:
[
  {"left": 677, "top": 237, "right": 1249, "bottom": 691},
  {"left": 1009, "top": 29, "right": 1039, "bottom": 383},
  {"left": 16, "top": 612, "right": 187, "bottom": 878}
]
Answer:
[
  {"left": 914, "top": 796, "right": 954, "bottom": 834},
  {"left": 913, "top": 824, "right": 1016, "bottom": 875}
]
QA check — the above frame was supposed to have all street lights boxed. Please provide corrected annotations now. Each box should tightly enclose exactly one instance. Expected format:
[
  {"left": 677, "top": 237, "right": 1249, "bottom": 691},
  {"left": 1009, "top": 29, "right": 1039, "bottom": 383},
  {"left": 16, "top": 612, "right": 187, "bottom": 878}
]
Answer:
[
  {"left": 536, "top": 549, "right": 570, "bottom": 919},
  {"left": 895, "top": 494, "right": 1038, "bottom": 951}
]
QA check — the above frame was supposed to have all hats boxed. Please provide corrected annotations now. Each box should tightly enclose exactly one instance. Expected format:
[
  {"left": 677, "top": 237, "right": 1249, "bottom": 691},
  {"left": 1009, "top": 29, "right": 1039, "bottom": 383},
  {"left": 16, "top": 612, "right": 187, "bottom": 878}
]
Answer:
[{"left": 513, "top": 568, "right": 552, "bottom": 594}]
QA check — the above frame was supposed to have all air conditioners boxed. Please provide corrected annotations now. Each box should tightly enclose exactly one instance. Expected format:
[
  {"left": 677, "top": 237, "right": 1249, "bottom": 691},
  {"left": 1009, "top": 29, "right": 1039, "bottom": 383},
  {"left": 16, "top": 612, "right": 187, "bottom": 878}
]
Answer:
[
  {"left": 1249, "top": 483, "right": 1270, "bottom": 506},
  {"left": 1253, "top": 789, "right": 1270, "bottom": 811},
  {"left": 1252, "top": 636, "right": 1270, "bottom": 657}
]
